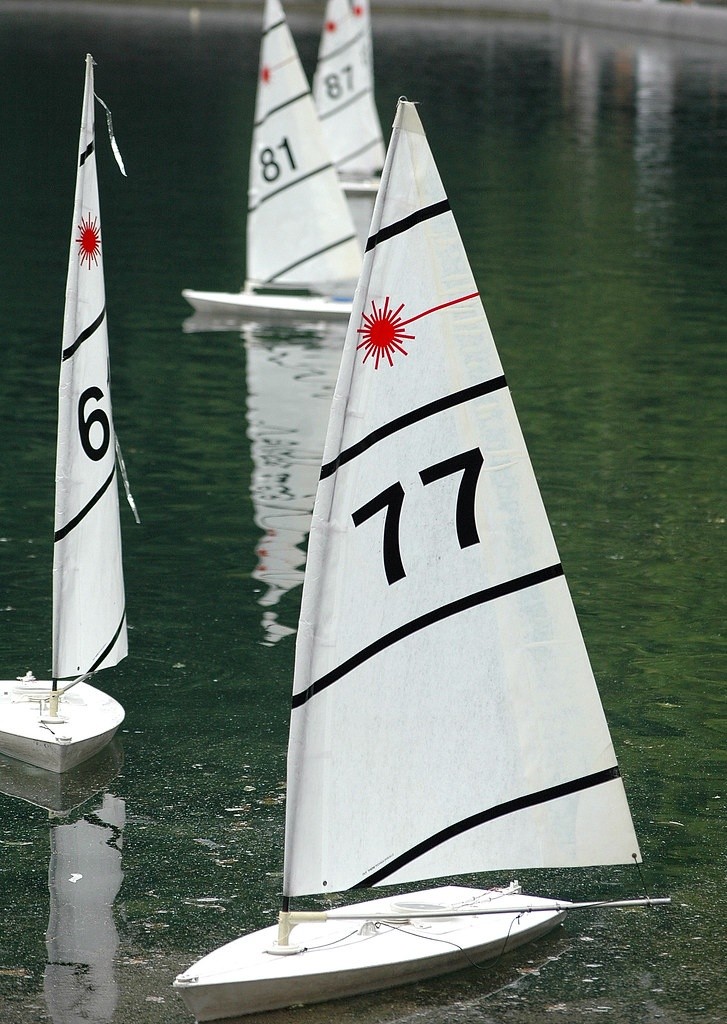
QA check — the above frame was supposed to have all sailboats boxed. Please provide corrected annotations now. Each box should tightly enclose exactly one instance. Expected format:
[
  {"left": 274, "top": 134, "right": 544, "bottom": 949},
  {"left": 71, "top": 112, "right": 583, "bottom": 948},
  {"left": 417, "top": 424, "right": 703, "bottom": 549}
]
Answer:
[
  {"left": 317, "top": 0, "right": 391, "bottom": 198},
  {"left": 182, "top": 307, "right": 357, "bottom": 643},
  {"left": 172, "top": 95, "right": 651, "bottom": 1024},
  {"left": 0, "top": 742, "right": 129, "bottom": 1024},
  {"left": 0, "top": 49, "right": 139, "bottom": 775},
  {"left": 181, "top": 0, "right": 366, "bottom": 323}
]
